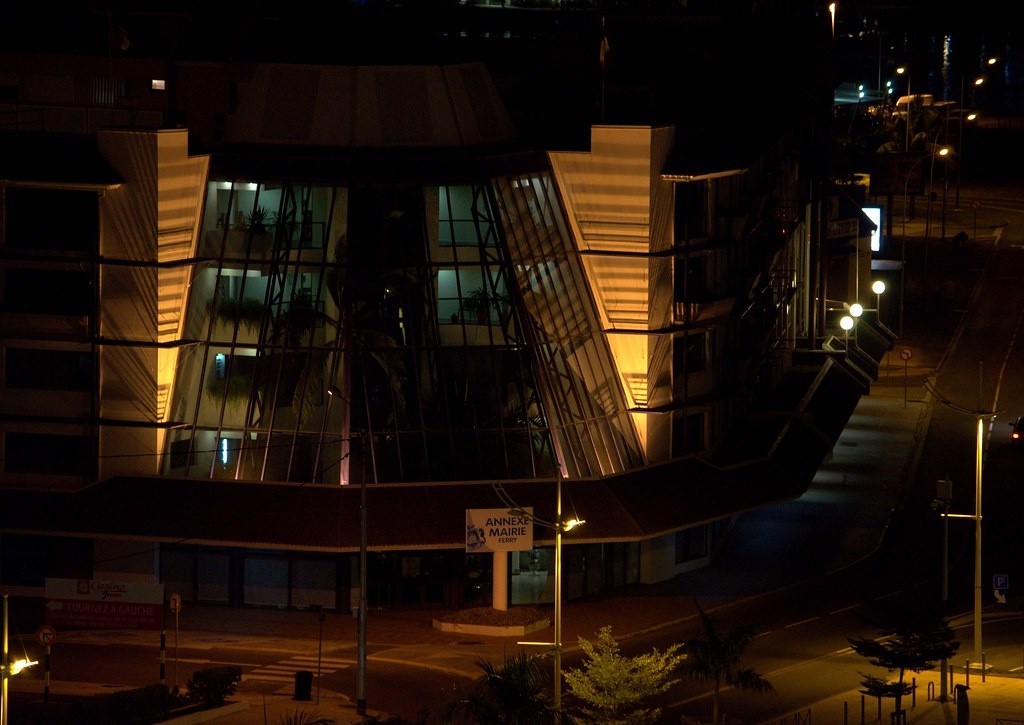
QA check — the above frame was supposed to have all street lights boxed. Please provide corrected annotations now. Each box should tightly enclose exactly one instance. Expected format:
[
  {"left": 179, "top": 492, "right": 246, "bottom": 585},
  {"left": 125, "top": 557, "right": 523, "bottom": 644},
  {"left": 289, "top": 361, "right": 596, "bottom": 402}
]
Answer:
[
  {"left": 490, "top": 467, "right": 585, "bottom": 725},
  {"left": 922, "top": 359, "right": 1007, "bottom": 675},
  {"left": 0, "top": 592, "right": 40, "bottom": 725},
  {"left": 922, "top": 111, "right": 978, "bottom": 280},
  {"left": 897, "top": 66, "right": 912, "bottom": 156},
  {"left": 934, "top": 476, "right": 954, "bottom": 701},
  {"left": 326, "top": 385, "right": 372, "bottom": 719},
  {"left": 955, "top": 71, "right": 984, "bottom": 211},
  {"left": 895, "top": 147, "right": 952, "bottom": 342}
]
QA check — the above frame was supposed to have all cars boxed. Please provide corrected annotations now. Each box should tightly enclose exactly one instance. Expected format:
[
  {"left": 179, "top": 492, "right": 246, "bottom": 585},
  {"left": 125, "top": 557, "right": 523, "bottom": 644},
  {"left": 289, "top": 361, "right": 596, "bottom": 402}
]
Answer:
[{"left": 1008, "top": 416, "right": 1024, "bottom": 449}]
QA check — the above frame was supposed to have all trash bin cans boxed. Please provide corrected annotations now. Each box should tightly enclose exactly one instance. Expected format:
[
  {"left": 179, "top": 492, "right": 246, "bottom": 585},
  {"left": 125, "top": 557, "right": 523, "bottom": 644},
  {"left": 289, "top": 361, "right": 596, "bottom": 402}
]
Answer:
[{"left": 293, "top": 671, "right": 313, "bottom": 701}]
